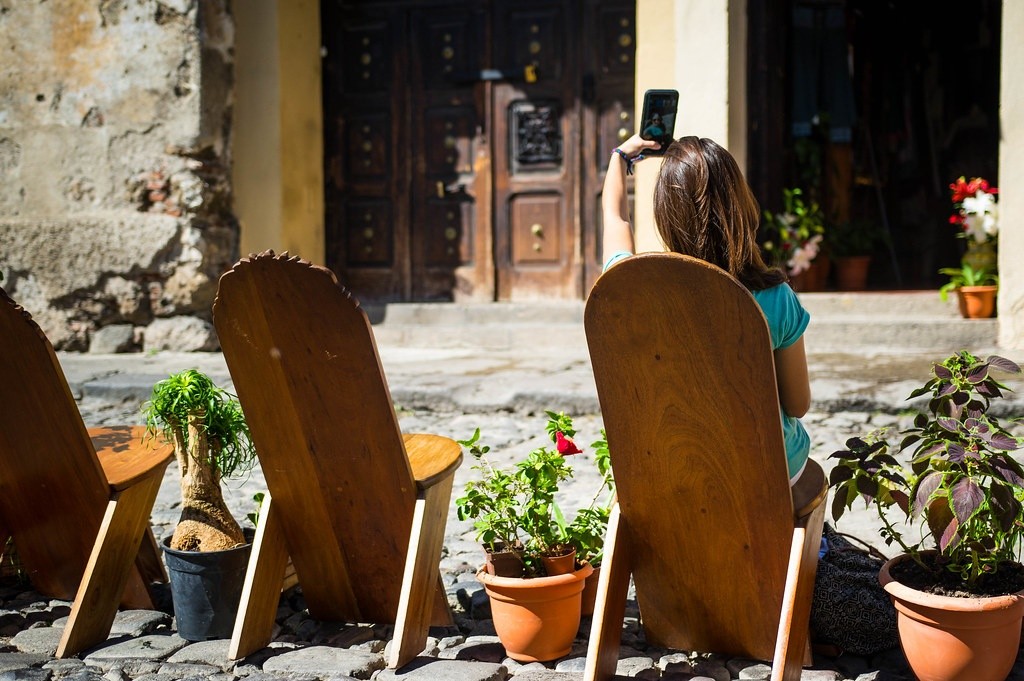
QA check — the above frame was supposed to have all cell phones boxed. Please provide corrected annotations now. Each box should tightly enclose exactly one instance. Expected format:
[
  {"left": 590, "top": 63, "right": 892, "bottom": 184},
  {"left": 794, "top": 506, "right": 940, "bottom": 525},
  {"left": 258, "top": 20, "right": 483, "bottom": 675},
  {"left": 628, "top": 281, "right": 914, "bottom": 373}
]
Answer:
[{"left": 639, "top": 89, "right": 679, "bottom": 155}]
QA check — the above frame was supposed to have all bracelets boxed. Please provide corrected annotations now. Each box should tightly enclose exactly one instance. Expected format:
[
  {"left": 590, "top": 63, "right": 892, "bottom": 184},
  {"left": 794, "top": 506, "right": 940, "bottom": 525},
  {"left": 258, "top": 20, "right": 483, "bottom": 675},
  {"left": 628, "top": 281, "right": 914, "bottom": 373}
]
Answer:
[{"left": 607, "top": 147, "right": 645, "bottom": 175}]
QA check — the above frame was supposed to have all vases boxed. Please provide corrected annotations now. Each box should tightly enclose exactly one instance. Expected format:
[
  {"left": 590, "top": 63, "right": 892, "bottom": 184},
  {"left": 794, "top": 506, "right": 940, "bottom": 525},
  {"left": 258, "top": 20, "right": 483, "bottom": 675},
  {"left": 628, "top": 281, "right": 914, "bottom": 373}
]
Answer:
[
  {"left": 961, "top": 241, "right": 997, "bottom": 275},
  {"left": 474, "top": 559, "right": 593, "bottom": 663},
  {"left": 791, "top": 248, "right": 830, "bottom": 292}
]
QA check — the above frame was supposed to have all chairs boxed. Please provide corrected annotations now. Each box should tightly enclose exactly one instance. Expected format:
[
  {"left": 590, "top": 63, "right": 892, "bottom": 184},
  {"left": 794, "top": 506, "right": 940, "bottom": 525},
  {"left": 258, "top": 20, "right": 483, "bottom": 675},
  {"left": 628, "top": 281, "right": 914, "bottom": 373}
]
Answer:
[
  {"left": 582, "top": 252, "right": 829, "bottom": 681},
  {"left": 212, "top": 247, "right": 464, "bottom": 669},
  {"left": 0, "top": 287, "right": 177, "bottom": 660}
]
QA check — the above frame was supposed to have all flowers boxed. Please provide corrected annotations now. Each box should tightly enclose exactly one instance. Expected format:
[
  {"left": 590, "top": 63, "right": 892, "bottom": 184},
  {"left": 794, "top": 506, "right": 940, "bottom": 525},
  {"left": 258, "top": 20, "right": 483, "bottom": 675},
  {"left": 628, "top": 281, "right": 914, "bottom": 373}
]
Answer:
[
  {"left": 949, "top": 176, "right": 999, "bottom": 244},
  {"left": 762, "top": 187, "right": 826, "bottom": 278},
  {"left": 455, "top": 409, "right": 583, "bottom": 578}
]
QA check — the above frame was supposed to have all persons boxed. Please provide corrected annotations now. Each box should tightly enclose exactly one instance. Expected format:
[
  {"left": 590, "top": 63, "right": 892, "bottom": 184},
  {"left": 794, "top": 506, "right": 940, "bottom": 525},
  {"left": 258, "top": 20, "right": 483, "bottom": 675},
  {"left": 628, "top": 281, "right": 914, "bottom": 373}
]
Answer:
[
  {"left": 641, "top": 112, "right": 667, "bottom": 138},
  {"left": 600, "top": 133, "right": 813, "bottom": 486}
]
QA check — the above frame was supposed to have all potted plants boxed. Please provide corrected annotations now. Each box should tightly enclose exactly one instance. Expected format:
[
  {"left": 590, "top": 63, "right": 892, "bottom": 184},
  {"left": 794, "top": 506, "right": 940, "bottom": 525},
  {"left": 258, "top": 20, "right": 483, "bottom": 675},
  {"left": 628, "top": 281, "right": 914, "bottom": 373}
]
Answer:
[
  {"left": 937, "top": 264, "right": 999, "bottom": 318},
  {"left": 824, "top": 349, "right": 1024, "bottom": 681},
  {"left": 825, "top": 220, "right": 894, "bottom": 292},
  {"left": 574, "top": 428, "right": 614, "bottom": 617},
  {"left": 525, "top": 502, "right": 576, "bottom": 575},
  {"left": 137, "top": 368, "right": 264, "bottom": 642},
  {"left": 472, "top": 513, "right": 524, "bottom": 577}
]
